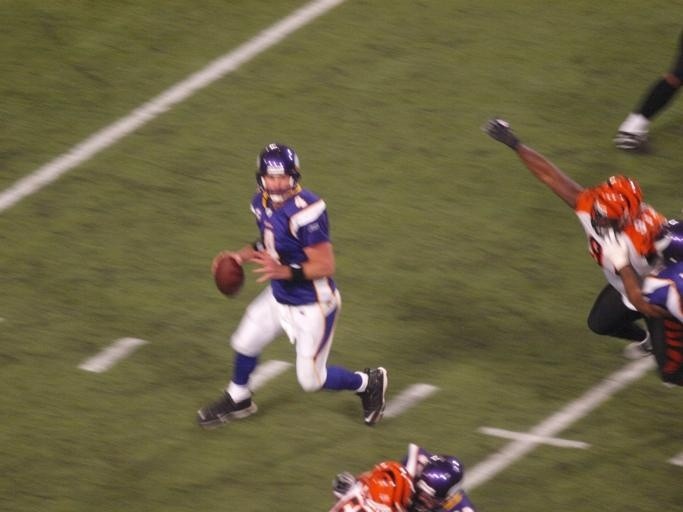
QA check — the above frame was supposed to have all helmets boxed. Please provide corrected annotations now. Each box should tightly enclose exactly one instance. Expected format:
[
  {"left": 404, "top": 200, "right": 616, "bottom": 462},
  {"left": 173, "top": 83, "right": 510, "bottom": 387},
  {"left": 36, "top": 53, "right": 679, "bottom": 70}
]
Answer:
[
  {"left": 417, "top": 454, "right": 463, "bottom": 498},
  {"left": 655, "top": 220, "right": 683, "bottom": 266},
  {"left": 589, "top": 175, "right": 644, "bottom": 236},
  {"left": 254, "top": 143, "right": 301, "bottom": 202},
  {"left": 362, "top": 462, "right": 414, "bottom": 511}
]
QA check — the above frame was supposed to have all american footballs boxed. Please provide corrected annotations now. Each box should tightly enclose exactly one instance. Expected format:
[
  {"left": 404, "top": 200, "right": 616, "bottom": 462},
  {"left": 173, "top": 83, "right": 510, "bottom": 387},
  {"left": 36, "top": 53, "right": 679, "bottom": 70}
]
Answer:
[{"left": 214, "top": 255, "right": 245, "bottom": 299}]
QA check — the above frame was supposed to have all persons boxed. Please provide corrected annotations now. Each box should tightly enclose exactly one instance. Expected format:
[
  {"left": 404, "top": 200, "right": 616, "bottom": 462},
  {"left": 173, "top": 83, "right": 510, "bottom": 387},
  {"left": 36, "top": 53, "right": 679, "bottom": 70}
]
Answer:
[
  {"left": 643, "top": 216, "right": 683, "bottom": 466},
  {"left": 196, "top": 145, "right": 386, "bottom": 425},
  {"left": 612, "top": 32, "right": 682, "bottom": 149},
  {"left": 331, "top": 461, "right": 414, "bottom": 511},
  {"left": 331, "top": 443, "right": 477, "bottom": 511},
  {"left": 484, "top": 118, "right": 668, "bottom": 358}
]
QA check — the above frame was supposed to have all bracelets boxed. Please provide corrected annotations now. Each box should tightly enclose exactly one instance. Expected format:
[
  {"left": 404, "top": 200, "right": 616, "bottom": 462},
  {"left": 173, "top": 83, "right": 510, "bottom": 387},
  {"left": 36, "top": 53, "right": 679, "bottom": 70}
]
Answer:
[{"left": 289, "top": 260, "right": 304, "bottom": 281}]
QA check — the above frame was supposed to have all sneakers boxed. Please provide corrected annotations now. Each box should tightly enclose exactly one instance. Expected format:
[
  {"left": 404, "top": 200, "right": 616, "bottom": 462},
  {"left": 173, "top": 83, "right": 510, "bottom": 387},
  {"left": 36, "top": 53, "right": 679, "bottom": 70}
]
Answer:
[
  {"left": 196, "top": 392, "right": 257, "bottom": 425},
  {"left": 356, "top": 366, "right": 388, "bottom": 425},
  {"left": 624, "top": 333, "right": 653, "bottom": 359},
  {"left": 615, "top": 131, "right": 649, "bottom": 149}
]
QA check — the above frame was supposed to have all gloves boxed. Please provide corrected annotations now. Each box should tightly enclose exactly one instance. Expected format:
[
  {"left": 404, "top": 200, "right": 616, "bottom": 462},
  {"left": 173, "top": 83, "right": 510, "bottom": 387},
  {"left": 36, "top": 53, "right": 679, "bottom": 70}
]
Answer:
[{"left": 480, "top": 120, "right": 518, "bottom": 148}]
